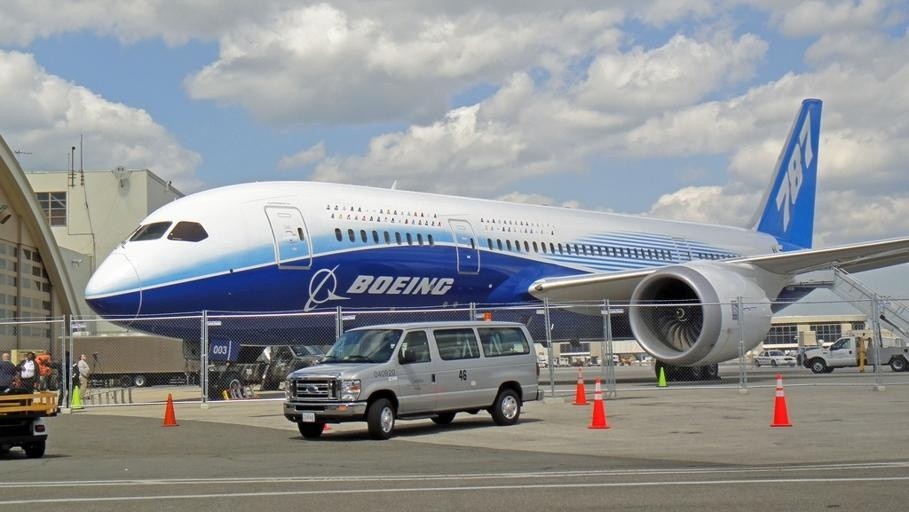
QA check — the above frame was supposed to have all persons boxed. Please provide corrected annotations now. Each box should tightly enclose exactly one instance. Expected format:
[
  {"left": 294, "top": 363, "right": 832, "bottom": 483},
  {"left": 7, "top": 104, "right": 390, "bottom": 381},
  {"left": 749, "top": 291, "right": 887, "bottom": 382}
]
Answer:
[{"left": 1, "top": 349, "right": 93, "bottom": 406}]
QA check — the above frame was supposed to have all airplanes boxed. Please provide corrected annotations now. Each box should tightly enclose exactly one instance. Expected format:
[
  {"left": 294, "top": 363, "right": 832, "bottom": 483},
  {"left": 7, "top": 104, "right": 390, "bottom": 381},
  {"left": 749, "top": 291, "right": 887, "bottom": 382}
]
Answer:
[{"left": 81, "top": 97, "right": 909, "bottom": 398}]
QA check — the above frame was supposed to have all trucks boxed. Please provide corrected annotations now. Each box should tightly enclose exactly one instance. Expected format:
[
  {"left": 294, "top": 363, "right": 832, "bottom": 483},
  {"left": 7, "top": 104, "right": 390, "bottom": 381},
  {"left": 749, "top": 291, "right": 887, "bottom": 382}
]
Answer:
[{"left": 45, "top": 332, "right": 203, "bottom": 389}]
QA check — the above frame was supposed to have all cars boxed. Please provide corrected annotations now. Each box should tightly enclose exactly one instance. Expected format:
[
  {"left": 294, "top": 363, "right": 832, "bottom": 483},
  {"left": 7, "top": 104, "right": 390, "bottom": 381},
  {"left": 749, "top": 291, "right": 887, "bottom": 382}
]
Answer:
[
  {"left": 540, "top": 352, "right": 655, "bottom": 367},
  {"left": 212, "top": 336, "right": 326, "bottom": 389},
  {"left": 751, "top": 346, "right": 798, "bottom": 369}
]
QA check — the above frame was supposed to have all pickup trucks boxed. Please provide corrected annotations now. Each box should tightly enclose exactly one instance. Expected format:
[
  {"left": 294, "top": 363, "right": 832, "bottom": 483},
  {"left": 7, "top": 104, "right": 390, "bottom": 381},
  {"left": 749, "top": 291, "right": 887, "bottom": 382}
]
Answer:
[{"left": 802, "top": 335, "right": 909, "bottom": 376}]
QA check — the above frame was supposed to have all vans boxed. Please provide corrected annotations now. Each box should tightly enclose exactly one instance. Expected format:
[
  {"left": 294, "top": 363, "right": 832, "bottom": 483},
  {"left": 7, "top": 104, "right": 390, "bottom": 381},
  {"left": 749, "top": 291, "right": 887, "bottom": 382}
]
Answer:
[{"left": 282, "top": 317, "right": 547, "bottom": 444}]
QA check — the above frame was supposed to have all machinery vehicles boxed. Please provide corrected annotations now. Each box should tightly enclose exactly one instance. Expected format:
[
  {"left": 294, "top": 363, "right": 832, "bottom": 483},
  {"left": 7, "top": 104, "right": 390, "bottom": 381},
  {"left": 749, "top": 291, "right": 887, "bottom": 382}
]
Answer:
[{"left": 0, "top": 390, "right": 61, "bottom": 459}]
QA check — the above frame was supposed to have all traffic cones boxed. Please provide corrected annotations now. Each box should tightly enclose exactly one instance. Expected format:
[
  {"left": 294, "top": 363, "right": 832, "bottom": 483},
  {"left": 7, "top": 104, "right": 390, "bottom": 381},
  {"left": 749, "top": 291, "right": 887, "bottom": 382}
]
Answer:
[
  {"left": 655, "top": 365, "right": 670, "bottom": 387},
  {"left": 585, "top": 386, "right": 612, "bottom": 429},
  {"left": 71, "top": 384, "right": 85, "bottom": 409},
  {"left": 323, "top": 423, "right": 332, "bottom": 430},
  {"left": 572, "top": 366, "right": 591, "bottom": 405},
  {"left": 769, "top": 372, "right": 796, "bottom": 428},
  {"left": 158, "top": 392, "right": 180, "bottom": 427}
]
